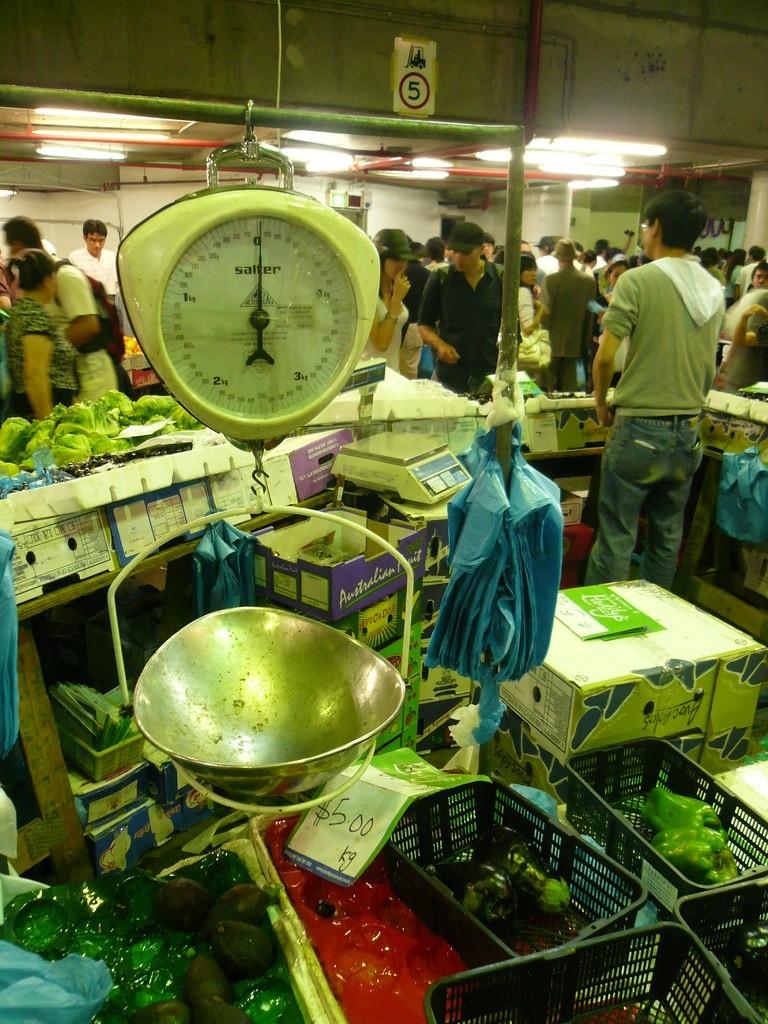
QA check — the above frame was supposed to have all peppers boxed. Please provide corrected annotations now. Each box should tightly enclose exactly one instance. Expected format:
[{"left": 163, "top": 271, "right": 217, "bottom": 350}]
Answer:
[{"left": 639, "top": 785, "right": 739, "bottom": 886}]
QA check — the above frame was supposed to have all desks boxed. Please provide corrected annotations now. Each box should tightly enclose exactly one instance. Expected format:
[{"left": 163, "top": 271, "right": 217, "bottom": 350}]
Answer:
[
  {"left": 672, "top": 445, "right": 721, "bottom": 598},
  {"left": 19, "top": 486, "right": 337, "bottom": 883}
]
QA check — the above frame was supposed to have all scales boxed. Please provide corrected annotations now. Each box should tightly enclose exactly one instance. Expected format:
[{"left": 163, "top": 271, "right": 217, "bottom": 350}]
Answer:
[
  {"left": 330, "top": 357, "right": 473, "bottom": 505},
  {"left": 108, "top": 100, "right": 414, "bottom": 816}
]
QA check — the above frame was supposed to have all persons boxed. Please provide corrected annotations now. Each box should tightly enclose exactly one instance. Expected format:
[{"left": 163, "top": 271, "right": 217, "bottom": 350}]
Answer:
[
  {"left": 585, "top": 188, "right": 725, "bottom": 590},
  {"left": 362, "top": 221, "right": 768, "bottom": 396},
  {"left": 0, "top": 216, "right": 136, "bottom": 420}
]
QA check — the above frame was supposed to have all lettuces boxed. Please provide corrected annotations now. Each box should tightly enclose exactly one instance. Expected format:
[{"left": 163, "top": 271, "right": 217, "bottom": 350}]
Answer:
[{"left": 0, "top": 389, "right": 206, "bottom": 482}]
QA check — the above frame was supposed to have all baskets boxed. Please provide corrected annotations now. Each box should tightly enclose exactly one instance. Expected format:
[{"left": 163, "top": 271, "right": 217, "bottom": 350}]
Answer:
[{"left": 53, "top": 726, "right": 147, "bottom": 781}]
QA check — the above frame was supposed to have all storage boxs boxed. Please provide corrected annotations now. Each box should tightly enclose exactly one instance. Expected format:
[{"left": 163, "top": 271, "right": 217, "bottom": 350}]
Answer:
[{"left": 0, "top": 349, "right": 768, "bottom": 1024}]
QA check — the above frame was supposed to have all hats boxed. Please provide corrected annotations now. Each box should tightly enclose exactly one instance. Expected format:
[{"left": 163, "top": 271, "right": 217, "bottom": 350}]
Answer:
[
  {"left": 551, "top": 238, "right": 581, "bottom": 261},
  {"left": 373, "top": 229, "right": 423, "bottom": 260},
  {"left": 446, "top": 222, "right": 484, "bottom": 251}
]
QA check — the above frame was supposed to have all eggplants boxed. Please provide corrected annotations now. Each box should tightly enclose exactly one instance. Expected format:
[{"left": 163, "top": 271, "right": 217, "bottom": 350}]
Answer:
[{"left": 421, "top": 834, "right": 571, "bottom": 929}]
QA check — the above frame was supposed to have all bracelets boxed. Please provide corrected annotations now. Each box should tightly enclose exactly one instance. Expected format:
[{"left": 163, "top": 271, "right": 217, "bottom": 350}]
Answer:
[{"left": 385, "top": 312, "right": 397, "bottom": 325}]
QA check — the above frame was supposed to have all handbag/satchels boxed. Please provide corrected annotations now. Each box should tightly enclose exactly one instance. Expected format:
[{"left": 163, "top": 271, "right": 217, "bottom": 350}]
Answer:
[{"left": 518, "top": 330, "right": 551, "bottom": 370}]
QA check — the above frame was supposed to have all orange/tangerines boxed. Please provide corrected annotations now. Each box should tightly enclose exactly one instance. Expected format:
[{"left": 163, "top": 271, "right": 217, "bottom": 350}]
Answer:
[{"left": 123, "top": 335, "right": 141, "bottom": 357}]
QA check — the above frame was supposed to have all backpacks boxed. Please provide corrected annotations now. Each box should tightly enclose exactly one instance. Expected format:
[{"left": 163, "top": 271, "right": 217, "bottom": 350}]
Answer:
[{"left": 53, "top": 259, "right": 125, "bottom": 360}]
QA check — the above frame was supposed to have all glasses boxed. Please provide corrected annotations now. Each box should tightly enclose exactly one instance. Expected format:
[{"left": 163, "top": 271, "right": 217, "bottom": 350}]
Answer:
[
  {"left": 641, "top": 223, "right": 649, "bottom": 231},
  {"left": 89, "top": 239, "right": 105, "bottom": 243}
]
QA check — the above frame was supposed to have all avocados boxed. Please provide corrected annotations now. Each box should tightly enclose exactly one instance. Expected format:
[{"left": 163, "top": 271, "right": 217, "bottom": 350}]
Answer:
[{"left": 127, "top": 876, "right": 275, "bottom": 1024}]
